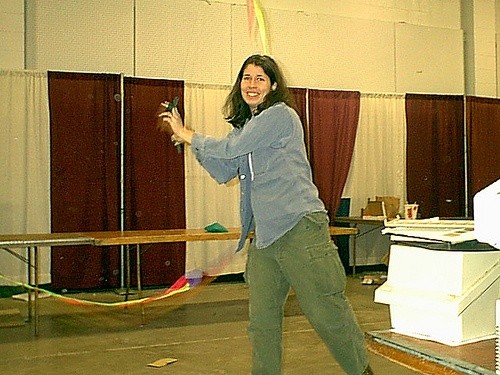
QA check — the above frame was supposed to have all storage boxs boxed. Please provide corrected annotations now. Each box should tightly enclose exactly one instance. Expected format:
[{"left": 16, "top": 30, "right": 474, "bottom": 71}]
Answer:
[{"left": 388, "top": 239, "right": 500, "bottom": 346}]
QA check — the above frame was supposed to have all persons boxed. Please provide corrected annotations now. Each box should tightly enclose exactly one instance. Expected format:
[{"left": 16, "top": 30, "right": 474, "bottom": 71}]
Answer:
[{"left": 158, "top": 54, "right": 373, "bottom": 375}]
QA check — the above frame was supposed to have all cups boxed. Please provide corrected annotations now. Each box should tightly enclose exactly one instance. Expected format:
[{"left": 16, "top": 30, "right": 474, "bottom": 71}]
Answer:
[{"left": 404, "top": 203, "right": 419, "bottom": 220}]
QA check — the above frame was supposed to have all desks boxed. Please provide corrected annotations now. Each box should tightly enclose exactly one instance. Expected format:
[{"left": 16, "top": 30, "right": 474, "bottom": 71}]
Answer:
[
  {"left": 337, "top": 216, "right": 392, "bottom": 278},
  {"left": 0, "top": 227, "right": 357, "bottom": 336}
]
